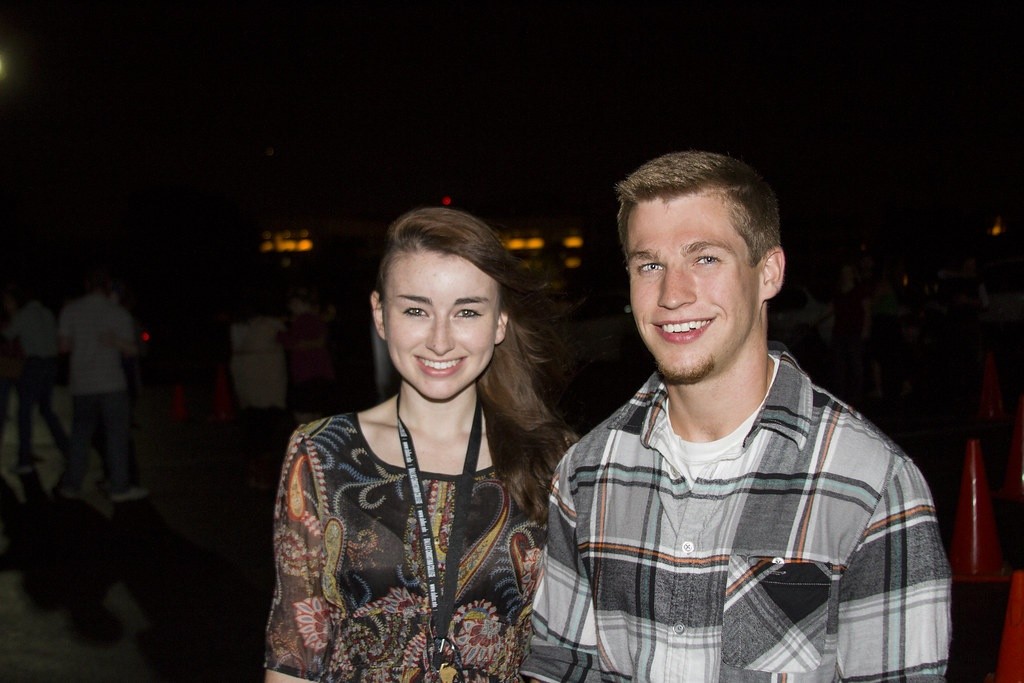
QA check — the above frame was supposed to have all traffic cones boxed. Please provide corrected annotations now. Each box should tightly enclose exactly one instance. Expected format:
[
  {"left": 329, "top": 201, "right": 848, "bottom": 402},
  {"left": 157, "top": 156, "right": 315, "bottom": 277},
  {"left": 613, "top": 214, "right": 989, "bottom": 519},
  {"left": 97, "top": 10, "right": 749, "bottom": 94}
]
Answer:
[
  {"left": 999, "top": 409, "right": 1023, "bottom": 501},
  {"left": 205, "top": 367, "right": 234, "bottom": 422},
  {"left": 978, "top": 352, "right": 1006, "bottom": 418},
  {"left": 170, "top": 380, "right": 191, "bottom": 422},
  {"left": 953, "top": 440, "right": 1008, "bottom": 581},
  {"left": 992, "top": 568, "right": 1024, "bottom": 682}
]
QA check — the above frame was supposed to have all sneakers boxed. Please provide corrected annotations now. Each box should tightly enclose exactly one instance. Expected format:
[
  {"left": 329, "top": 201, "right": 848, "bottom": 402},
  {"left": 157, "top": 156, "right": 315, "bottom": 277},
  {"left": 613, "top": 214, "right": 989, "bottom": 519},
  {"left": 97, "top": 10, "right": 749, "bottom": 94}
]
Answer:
[
  {"left": 110, "top": 486, "right": 147, "bottom": 502},
  {"left": 55, "top": 485, "right": 83, "bottom": 499},
  {"left": 10, "top": 459, "right": 36, "bottom": 474}
]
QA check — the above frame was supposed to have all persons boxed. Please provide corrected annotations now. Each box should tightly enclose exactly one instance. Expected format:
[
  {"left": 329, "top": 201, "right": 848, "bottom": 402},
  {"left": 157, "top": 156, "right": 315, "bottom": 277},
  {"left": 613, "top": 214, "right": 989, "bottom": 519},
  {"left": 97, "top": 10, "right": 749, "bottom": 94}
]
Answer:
[
  {"left": 0, "top": 265, "right": 343, "bottom": 502},
  {"left": 522, "top": 152, "right": 951, "bottom": 683},
  {"left": 263, "top": 209, "right": 581, "bottom": 683},
  {"left": 804, "top": 241, "right": 988, "bottom": 427}
]
